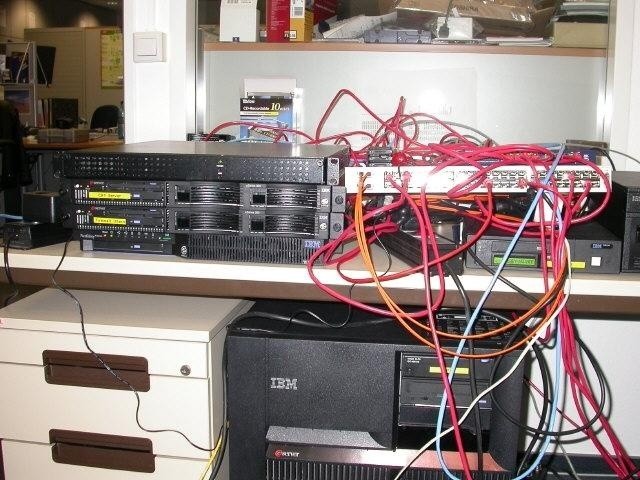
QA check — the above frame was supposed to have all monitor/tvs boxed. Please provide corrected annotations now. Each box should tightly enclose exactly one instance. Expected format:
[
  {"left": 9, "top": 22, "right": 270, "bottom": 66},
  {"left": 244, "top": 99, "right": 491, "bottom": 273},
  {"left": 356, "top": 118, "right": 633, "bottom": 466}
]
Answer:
[
  {"left": 51, "top": 97, "right": 78, "bottom": 129},
  {"left": 37, "top": 44, "right": 58, "bottom": 87}
]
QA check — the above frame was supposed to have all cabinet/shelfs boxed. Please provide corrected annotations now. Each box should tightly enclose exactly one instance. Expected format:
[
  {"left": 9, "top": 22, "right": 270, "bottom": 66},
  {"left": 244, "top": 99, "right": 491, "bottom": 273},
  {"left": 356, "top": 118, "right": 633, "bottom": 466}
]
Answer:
[{"left": 0, "top": 318, "right": 214, "bottom": 476}]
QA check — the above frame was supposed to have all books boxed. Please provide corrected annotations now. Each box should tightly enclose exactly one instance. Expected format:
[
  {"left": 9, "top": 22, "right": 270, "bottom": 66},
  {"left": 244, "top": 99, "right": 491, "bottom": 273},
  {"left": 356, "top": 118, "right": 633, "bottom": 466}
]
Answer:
[{"left": 484, "top": 0, "right": 611, "bottom": 48}]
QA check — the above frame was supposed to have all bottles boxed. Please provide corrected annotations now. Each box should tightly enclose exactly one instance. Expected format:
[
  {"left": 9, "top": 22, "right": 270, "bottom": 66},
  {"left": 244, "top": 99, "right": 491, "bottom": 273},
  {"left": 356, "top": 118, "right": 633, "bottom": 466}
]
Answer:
[{"left": 116, "top": 99, "right": 126, "bottom": 140}]
